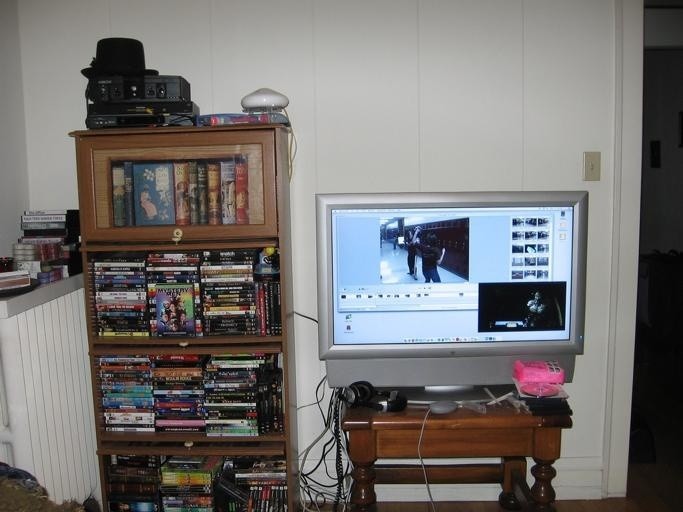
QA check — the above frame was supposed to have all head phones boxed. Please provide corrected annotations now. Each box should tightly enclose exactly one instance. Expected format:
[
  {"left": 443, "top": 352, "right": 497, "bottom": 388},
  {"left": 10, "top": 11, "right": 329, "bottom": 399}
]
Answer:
[{"left": 343, "top": 380, "right": 408, "bottom": 413}]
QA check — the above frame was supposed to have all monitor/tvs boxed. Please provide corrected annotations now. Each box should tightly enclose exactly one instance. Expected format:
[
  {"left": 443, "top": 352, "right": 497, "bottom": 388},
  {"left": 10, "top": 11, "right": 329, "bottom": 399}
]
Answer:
[{"left": 315, "top": 191, "right": 589, "bottom": 404}]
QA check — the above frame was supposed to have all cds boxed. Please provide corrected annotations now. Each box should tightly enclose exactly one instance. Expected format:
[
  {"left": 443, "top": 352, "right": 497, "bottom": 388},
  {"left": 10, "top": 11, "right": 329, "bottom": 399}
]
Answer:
[{"left": 521, "top": 384, "right": 559, "bottom": 397}]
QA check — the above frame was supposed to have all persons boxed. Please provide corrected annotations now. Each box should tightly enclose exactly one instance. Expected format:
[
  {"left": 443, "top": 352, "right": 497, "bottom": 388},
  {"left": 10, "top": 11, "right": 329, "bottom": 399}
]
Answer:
[
  {"left": 412, "top": 226, "right": 446, "bottom": 283},
  {"left": 405, "top": 230, "right": 419, "bottom": 277},
  {"left": 522, "top": 291, "right": 547, "bottom": 327}
]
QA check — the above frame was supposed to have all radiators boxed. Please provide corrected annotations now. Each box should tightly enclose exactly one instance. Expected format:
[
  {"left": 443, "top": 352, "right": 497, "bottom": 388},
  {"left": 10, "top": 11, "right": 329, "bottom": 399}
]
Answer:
[{"left": 1, "top": 272, "right": 103, "bottom": 511}]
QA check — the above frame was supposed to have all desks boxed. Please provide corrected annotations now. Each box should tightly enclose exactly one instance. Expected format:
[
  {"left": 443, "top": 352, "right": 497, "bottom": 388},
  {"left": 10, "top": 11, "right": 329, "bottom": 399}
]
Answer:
[{"left": 341, "top": 404, "right": 574, "bottom": 512}]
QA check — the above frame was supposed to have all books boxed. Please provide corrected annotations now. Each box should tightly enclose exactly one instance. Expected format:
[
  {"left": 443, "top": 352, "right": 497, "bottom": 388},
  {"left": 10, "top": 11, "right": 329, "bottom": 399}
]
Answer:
[
  {"left": 510, "top": 378, "right": 574, "bottom": 417},
  {"left": 18, "top": 208, "right": 78, "bottom": 243}
]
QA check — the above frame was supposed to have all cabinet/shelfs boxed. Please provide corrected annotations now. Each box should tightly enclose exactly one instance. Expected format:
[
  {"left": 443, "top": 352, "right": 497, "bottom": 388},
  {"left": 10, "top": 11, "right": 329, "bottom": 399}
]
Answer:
[{"left": 68, "top": 125, "right": 299, "bottom": 512}]
